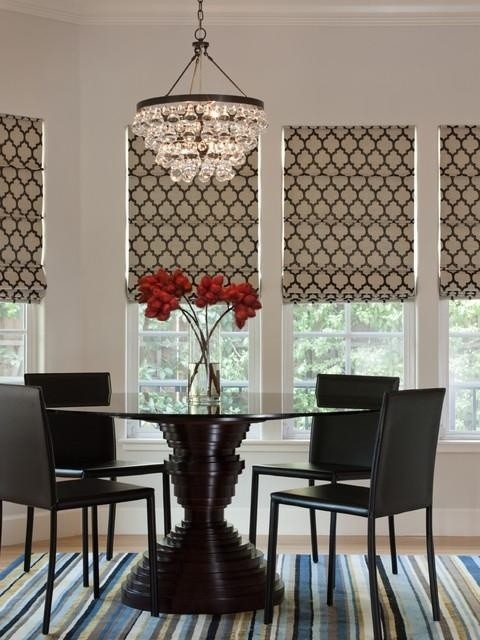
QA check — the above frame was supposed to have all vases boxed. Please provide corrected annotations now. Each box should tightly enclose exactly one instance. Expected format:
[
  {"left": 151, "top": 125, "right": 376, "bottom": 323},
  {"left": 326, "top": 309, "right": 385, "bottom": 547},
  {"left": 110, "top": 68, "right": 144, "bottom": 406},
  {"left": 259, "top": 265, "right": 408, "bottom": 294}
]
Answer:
[{"left": 188, "top": 361, "right": 221, "bottom": 406}]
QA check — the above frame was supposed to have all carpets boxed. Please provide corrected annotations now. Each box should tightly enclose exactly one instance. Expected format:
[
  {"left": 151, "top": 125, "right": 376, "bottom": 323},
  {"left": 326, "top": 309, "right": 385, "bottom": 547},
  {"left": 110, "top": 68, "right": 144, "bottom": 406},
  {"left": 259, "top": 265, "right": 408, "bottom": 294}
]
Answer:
[{"left": 1, "top": 550, "right": 480, "bottom": 640}]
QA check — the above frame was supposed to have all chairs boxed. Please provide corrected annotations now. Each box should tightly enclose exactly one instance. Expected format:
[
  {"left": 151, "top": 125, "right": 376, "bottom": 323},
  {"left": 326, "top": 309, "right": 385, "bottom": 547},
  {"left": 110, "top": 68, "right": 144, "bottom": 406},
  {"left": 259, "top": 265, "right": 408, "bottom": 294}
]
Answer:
[
  {"left": 24, "top": 373, "right": 171, "bottom": 587},
  {"left": 0, "top": 383, "right": 158, "bottom": 635},
  {"left": 264, "top": 388, "right": 446, "bottom": 639},
  {"left": 248, "top": 373, "right": 400, "bottom": 575}
]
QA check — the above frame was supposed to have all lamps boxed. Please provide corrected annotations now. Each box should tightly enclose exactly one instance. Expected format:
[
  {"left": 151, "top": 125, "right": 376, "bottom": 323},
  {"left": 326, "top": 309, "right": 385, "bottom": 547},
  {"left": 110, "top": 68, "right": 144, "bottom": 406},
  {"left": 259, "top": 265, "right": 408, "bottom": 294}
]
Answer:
[{"left": 131, "top": 0, "right": 268, "bottom": 187}]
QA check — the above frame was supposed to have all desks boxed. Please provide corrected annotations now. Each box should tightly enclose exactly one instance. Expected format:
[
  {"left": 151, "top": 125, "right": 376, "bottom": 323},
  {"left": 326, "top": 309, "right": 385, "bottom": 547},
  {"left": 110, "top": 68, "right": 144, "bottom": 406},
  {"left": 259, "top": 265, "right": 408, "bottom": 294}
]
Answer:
[{"left": 45, "top": 393, "right": 381, "bottom": 615}]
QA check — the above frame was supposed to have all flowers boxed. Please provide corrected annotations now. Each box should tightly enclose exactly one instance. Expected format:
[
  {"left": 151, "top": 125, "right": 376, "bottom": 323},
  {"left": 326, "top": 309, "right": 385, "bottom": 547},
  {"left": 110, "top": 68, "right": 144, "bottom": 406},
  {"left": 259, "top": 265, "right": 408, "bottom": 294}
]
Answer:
[{"left": 135, "top": 270, "right": 262, "bottom": 395}]
